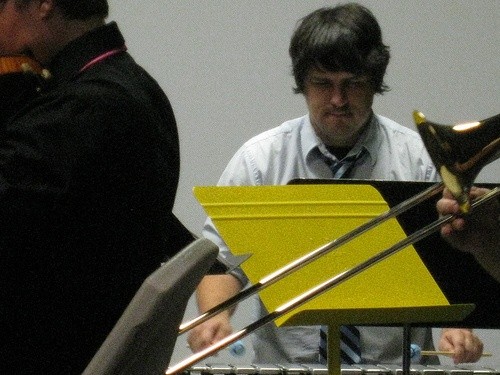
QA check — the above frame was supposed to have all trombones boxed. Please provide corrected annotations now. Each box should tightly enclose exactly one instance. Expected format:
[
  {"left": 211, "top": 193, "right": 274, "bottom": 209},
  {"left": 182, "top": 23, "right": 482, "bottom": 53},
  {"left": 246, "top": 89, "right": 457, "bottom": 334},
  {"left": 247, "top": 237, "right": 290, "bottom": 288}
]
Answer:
[{"left": 157, "top": 109, "right": 500, "bottom": 375}]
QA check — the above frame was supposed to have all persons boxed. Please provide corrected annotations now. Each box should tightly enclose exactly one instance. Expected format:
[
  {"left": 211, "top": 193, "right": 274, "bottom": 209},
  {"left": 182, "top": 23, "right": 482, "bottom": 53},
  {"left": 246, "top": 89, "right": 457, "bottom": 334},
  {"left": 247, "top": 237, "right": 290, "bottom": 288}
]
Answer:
[
  {"left": 437, "top": 184, "right": 499, "bottom": 284},
  {"left": 187, "top": 2, "right": 485, "bottom": 364},
  {"left": 1, "top": 0, "right": 181, "bottom": 375}
]
